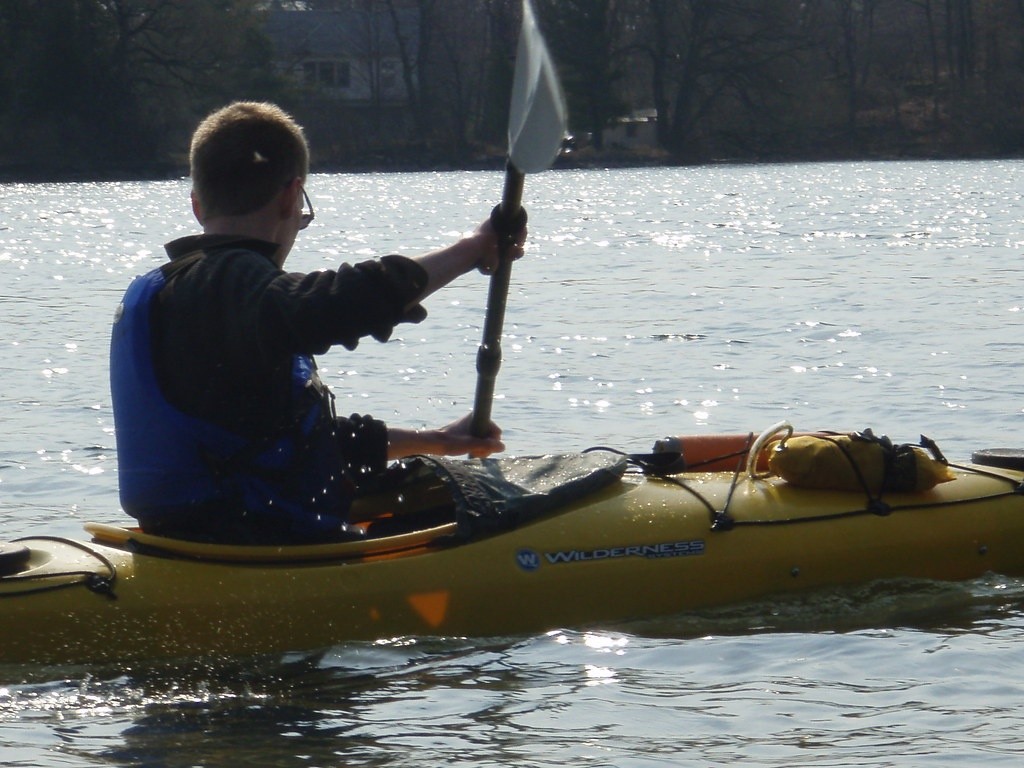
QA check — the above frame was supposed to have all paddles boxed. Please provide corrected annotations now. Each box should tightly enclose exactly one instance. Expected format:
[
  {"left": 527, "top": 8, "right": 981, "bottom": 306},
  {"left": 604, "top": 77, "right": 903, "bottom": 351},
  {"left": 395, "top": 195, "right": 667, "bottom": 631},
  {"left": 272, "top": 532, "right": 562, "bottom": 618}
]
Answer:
[{"left": 468, "top": 1, "right": 570, "bottom": 459}]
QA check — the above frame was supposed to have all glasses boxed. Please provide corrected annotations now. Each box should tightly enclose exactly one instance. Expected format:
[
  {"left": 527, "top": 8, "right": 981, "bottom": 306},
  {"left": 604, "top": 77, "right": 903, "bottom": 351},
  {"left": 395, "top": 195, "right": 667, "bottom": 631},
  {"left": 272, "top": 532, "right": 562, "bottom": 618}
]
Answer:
[{"left": 287, "top": 181, "right": 314, "bottom": 230}]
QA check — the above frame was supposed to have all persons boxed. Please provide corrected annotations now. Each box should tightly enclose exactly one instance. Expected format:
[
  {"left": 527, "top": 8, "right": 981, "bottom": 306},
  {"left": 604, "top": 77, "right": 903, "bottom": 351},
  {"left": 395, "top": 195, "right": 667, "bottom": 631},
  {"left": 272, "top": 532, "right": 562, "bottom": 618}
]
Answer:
[{"left": 111, "top": 99, "right": 528, "bottom": 545}]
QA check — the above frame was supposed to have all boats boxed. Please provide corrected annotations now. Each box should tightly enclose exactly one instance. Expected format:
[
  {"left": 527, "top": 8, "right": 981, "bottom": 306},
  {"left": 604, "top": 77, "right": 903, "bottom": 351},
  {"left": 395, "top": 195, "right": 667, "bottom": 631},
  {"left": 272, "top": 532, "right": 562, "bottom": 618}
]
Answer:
[{"left": 0, "top": 434, "right": 1024, "bottom": 656}]
